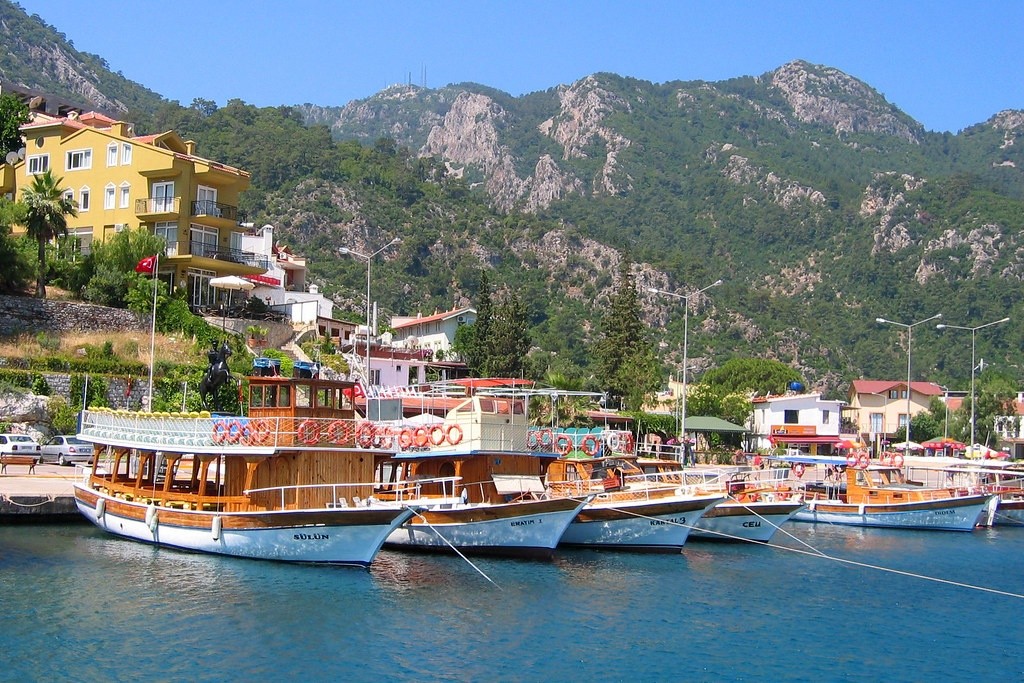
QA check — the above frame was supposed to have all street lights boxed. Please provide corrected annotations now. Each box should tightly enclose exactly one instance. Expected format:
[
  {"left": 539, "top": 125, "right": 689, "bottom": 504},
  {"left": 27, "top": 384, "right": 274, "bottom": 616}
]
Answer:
[
  {"left": 937, "top": 316, "right": 1011, "bottom": 461},
  {"left": 340, "top": 238, "right": 401, "bottom": 391},
  {"left": 675, "top": 366, "right": 697, "bottom": 461},
  {"left": 873, "top": 392, "right": 886, "bottom": 452},
  {"left": 931, "top": 383, "right": 947, "bottom": 439},
  {"left": 646, "top": 279, "right": 723, "bottom": 465},
  {"left": 876, "top": 314, "right": 943, "bottom": 457},
  {"left": 181, "top": 380, "right": 186, "bottom": 412}
]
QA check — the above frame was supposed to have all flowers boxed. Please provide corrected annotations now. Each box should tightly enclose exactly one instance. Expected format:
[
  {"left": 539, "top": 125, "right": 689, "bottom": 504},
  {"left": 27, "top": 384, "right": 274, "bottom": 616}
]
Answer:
[
  {"left": 667, "top": 438, "right": 680, "bottom": 445},
  {"left": 684, "top": 437, "right": 696, "bottom": 444},
  {"left": 769, "top": 441, "right": 792, "bottom": 469}
]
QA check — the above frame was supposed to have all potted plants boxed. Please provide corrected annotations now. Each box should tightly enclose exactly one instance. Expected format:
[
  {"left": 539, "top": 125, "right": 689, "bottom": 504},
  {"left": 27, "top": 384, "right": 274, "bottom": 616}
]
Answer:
[
  {"left": 246, "top": 324, "right": 261, "bottom": 348},
  {"left": 258, "top": 326, "right": 271, "bottom": 346}
]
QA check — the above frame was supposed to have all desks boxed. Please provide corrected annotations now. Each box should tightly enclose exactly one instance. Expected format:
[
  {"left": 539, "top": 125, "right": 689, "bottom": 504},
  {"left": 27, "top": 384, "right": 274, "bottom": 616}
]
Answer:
[{"left": 205, "top": 250, "right": 225, "bottom": 260}]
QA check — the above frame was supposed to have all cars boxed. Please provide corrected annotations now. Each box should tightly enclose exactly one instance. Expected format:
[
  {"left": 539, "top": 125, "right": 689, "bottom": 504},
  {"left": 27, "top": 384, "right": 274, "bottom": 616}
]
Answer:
[
  {"left": 0, "top": 433, "right": 40, "bottom": 465},
  {"left": 40, "top": 435, "right": 96, "bottom": 466}
]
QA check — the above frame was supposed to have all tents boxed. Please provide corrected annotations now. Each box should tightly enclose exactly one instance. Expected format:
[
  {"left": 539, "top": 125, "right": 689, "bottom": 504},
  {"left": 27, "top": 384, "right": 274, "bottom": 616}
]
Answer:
[{"left": 679, "top": 416, "right": 752, "bottom": 433}]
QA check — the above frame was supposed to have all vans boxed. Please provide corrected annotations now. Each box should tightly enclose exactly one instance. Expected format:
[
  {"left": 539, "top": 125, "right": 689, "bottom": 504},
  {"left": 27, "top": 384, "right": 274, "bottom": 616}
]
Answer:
[{"left": 771, "top": 449, "right": 806, "bottom": 467}]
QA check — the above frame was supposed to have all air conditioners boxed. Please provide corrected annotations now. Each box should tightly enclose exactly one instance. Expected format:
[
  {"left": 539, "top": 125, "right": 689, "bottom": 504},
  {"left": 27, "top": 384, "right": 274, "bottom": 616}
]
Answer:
[
  {"left": 115, "top": 223, "right": 128, "bottom": 233},
  {"left": 456, "top": 315, "right": 466, "bottom": 322}
]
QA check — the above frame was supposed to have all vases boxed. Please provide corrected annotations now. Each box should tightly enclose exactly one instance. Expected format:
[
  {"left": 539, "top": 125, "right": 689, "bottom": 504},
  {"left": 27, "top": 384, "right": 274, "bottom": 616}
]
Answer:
[{"left": 773, "top": 470, "right": 789, "bottom": 479}]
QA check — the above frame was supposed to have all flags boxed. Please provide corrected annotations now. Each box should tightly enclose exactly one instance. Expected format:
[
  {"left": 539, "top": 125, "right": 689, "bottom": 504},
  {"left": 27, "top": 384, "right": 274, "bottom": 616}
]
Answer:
[{"left": 135, "top": 256, "right": 157, "bottom": 272}]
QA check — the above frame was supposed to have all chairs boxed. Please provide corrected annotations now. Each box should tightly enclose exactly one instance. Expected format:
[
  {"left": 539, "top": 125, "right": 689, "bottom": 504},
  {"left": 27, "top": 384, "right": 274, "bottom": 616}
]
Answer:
[
  {"left": 196, "top": 204, "right": 205, "bottom": 215},
  {"left": 352, "top": 496, "right": 364, "bottom": 508},
  {"left": 214, "top": 208, "right": 223, "bottom": 218},
  {"left": 339, "top": 497, "right": 349, "bottom": 507}
]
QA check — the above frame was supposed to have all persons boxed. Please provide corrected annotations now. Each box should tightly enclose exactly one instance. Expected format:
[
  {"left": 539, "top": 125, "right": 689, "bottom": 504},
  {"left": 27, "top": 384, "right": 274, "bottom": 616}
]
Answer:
[
  {"left": 754, "top": 453, "right": 761, "bottom": 470},
  {"left": 207, "top": 340, "right": 231, "bottom": 384}
]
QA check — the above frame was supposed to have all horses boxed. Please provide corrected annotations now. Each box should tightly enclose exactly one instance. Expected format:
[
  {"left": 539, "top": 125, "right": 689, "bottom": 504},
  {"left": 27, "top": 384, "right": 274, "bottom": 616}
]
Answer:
[{"left": 195, "top": 340, "right": 239, "bottom": 412}]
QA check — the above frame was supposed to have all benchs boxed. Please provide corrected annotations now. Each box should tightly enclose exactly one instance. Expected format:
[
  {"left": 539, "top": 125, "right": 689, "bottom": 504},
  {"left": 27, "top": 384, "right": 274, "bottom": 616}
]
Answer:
[
  {"left": 99, "top": 477, "right": 223, "bottom": 512},
  {"left": 0, "top": 456, "right": 39, "bottom": 475}
]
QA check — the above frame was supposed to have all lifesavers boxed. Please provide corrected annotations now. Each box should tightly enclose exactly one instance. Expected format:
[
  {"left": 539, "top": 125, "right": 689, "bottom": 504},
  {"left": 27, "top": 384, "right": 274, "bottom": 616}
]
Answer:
[
  {"left": 373, "top": 426, "right": 394, "bottom": 449},
  {"left": 793, "top": 462, "right": 805, "bottom": 477},
  {"left": 356, "top": 422, "right": 375, "bottom": 448},
  {"left": 880, "top": 452, "right": 904, "bottom": 467},
  {"left": 731, "top": 485, "right": 757, "bottom": 502},
  {"left": 254, "top": 420, "right": 269, "bottom": 444},
  {"left": 827, "top": 466, "right": 839, "bottom": 483},
  {"left": 398, "top": 429, "right": 413, "bottom": 448},
  {"left": 212, "top": 422, "right": 226, "bottom": 442},
  {"left": 226, "top": 421, "right": 240, "bottom": 443},
  {"left": 240, "top": 424, "right": 254, "bottom": 445},
  {"left": 847, "top": 453, "right": 869, "bottom": 468},
  {"left": 527, "top": 429, "right": 620, "bottom": 456},
  {"left": 989, "top": 474, "right": 1002, "bottom": 482},
  {"left": 446, "top": 424, "right": 463, "bottom": 445},
  {"left": 328, "top": 421, "right": 350, "bottom": 444},
  {"left": 298, "top": 420, "right": 320, "bottom": 445},
  {"left": 428, "top": 425, "right": 445, "bottom": 445},
  {"left": 775, "top": 484, "right": 789, "bottom": 501},
  {"left": 413, "top": 426, "right": 428, "bottom": 447}
]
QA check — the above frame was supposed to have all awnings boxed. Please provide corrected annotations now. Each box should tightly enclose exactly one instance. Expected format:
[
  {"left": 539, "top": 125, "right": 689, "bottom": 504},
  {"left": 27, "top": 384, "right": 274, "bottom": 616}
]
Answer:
[
  {"left": 773, "top": 437, "right": 843, "bottom": 443},
  {"left": 242, "top": 274, "right": 280, "bottom": 287}
]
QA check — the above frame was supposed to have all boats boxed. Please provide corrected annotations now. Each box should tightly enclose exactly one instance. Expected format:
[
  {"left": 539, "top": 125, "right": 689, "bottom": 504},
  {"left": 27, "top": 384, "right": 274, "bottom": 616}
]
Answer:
[
  {"left": 639, "top": 457, "right": 810, "bottom": 545},
  {"left": 542, "top": 452, "right": 728, "bottom": 555},
  {"left": 354, "top": 377, "right": 599, "bottom": 559},
  {"left": 73, "top": 357, "right": 461, "bottom": 567},
  {"left": 769, "top": 454, "right": 1024, "bottom": 533}
]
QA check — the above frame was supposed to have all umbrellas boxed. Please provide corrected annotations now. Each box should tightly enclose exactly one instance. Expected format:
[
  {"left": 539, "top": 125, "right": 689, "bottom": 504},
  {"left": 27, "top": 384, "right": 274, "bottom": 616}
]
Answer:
[
  {"left": 210, "top": 275, "right": 256, "bottom": 317},
  {"left": 965, "top": 443, "right": 1007, "bottom": 460},
  {"left": 892, "top": 441, "right": 923, "bottom": 455},
  {"left": 920, "top": 437, "right": 966, "bottom": 456}
]
